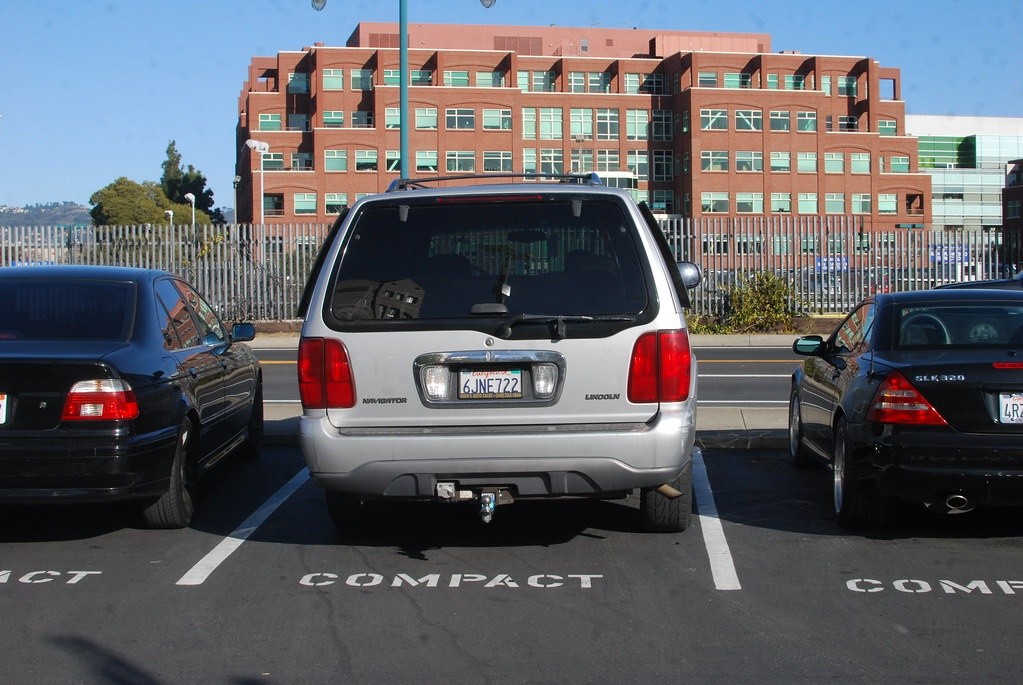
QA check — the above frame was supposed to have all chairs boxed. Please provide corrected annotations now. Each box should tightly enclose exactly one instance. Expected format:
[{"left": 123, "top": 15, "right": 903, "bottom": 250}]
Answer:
[{"left": 418, "top": 249, "right": 629, "bottom": 315}]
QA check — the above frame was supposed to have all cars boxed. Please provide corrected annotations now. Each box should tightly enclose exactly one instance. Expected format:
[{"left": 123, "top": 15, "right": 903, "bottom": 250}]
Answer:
[
  {"left": 790, "top": 271, "right": 1023, "bottom": 525},
  {"left": 0, "top": 265, "right": 265, "bottom": 532}
]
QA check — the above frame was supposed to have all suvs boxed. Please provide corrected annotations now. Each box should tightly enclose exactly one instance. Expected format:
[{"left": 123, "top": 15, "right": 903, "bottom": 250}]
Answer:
[{"left": 296, "top": 174, "right": 700, "bottom": 533}]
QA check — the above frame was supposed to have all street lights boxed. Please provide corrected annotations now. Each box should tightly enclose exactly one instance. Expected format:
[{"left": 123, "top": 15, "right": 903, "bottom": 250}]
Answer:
[
  {"left": 164, "top": 209, "right": 175, "bottom": 271},
  {"left": 245, "top": 138, "right": 269, "bottom": 308},
  {"left": 184, "top": 193, "right": 196, "bottom": 292}
]
